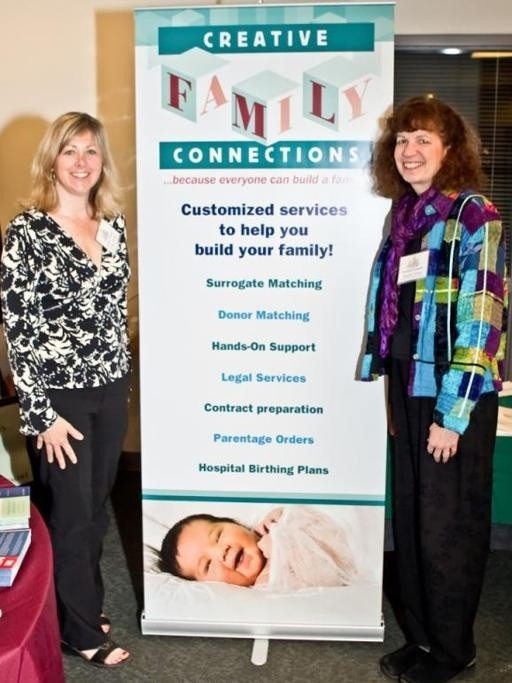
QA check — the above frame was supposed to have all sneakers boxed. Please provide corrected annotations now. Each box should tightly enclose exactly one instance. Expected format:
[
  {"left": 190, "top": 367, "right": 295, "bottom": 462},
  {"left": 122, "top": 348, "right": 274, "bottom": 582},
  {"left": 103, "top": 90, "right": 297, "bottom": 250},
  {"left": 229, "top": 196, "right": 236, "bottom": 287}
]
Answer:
[
  {"left": 399, "top": 643, "right": 477, "bottom": 683},
  {"left": 379, "top": 641, "right": 430, "bottom": 679}
]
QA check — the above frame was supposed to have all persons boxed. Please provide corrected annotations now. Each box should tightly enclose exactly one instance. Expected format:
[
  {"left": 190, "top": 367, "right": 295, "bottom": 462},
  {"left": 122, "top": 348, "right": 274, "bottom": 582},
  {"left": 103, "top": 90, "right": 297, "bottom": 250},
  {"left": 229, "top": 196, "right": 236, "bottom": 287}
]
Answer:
[
  {"left": 158, "top": 505, "right": 355, "bottom": 591},
  {"left": 0, "top": 113, "right": 132, "bottom": 668},
  {"left": 361, "top": 96, "right": 508, "bottom": 683}
]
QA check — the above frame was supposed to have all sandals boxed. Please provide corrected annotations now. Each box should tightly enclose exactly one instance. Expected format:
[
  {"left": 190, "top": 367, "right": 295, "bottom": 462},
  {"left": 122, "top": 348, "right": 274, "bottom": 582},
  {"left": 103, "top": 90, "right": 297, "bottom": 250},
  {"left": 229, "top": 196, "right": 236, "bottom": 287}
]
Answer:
[
  {"left": 100, "top": 610, "right": 113, "bottom": 634},
  {"left": 60, "top": 631, "right": 134, "bottom": 666}
]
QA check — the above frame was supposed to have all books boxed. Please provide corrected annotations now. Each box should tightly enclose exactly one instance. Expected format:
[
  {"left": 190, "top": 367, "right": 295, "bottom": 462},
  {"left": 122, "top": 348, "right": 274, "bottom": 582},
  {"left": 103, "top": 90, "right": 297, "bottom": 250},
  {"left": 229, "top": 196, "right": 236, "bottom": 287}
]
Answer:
[{"left": 0, "top": 486, "right": 31, "bottom": 587}]
